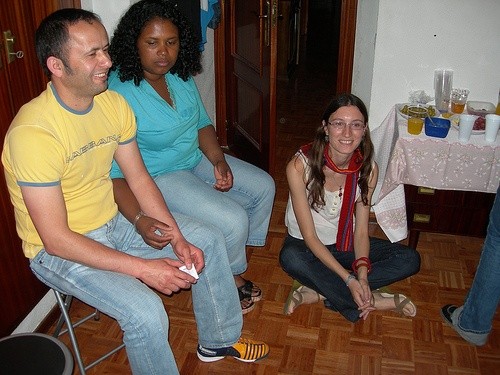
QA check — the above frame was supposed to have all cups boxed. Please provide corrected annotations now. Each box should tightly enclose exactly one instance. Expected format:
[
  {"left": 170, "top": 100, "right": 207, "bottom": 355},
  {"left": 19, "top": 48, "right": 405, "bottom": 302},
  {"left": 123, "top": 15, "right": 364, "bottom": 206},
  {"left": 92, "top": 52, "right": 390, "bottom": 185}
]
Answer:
[
  {"left": 485, "top": 114, "right": 500, "bottom": 144},
  {"left": 459, "top": 113, "right": 480, "bottom": 141},
  {"left": 408, "top": 106, "right": 428, "bottom": 135},
  {"left": 451, "top": 88, "right": 469, "bottom": 113},
  {"left": 433, "top": 70, "right": 452, "bottom": 112},
  {"left": 496, "top": 90, "right": 500, "bottom": 116}
]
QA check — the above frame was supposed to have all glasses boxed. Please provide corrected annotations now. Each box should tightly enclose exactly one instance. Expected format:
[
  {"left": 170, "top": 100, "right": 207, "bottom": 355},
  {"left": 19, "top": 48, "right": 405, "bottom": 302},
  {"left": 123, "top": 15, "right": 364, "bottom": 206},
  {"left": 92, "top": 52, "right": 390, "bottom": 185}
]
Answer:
[{"left": 328, "top": 119, "right": 366, "bottom": 130}]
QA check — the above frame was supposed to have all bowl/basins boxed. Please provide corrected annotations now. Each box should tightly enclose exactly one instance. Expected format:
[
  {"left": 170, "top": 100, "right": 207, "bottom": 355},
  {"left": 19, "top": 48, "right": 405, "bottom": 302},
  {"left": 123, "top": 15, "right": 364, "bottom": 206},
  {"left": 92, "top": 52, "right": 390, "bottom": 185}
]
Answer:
[
  {"left": 425, "top": 117, "right": 451, "bottom": 137},
  {"left": 467, "top": 100, "right": 497, "bottom": 117}
]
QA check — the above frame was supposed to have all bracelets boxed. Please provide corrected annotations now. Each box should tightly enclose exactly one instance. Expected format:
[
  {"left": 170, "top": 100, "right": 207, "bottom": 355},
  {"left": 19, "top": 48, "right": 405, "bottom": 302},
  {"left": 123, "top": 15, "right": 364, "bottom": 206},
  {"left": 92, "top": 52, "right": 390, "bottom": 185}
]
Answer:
[
  {"left": 352, "top": 257, "right": 371, "bottom": 275},
  {"left": 135, "top": 213, "right": 141, "bottom": 221},
  {"left": 345, "top": 275, "right": 356, "bottom": 286}
]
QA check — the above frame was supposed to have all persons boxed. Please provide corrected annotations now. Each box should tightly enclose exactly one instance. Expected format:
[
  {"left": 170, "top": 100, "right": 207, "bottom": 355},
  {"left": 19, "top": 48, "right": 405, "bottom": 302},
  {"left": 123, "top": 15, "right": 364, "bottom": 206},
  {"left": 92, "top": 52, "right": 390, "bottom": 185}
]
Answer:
[
  {"left": 106, "top": 0, "right": 276, "bottom": 313},
  {"left": 440, "top": 181, "right": 500, "bottom": 345},
  {"left": 279, "top": 93, "right": 421, "bottom": 323},
  {"left": 1, "top": 7, "right": 270, "bottom": 375}
]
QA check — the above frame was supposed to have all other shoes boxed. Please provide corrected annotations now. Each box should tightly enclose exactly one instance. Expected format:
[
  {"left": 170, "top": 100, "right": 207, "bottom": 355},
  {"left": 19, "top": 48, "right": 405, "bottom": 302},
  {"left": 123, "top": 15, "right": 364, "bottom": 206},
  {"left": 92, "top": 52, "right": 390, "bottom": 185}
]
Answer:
[{"left": 440, "top": 304, "right": 458, "bottom": 325}]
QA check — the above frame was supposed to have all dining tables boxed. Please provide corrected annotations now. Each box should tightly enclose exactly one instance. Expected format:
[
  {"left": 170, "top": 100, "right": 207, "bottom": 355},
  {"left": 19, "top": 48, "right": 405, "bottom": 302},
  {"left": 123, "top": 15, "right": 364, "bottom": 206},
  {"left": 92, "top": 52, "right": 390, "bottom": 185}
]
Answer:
[{"left": 372, "top": 103, "right": 500, "bottom": 250}]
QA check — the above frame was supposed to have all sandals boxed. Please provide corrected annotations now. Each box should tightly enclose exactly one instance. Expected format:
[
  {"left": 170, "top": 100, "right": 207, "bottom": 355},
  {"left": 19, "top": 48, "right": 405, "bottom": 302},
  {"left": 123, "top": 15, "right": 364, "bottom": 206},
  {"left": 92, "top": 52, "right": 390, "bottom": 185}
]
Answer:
[
  {"left": 234, "top": 288, "right": 256, "bottom": 314},
  {"left": 239, "top": 280, "right": 262, "bottom": 302}
]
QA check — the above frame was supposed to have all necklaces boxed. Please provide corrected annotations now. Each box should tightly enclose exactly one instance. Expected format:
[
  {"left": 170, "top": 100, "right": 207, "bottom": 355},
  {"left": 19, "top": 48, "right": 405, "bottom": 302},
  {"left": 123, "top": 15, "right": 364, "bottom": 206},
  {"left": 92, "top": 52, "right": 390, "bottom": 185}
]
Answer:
[{"left": 334, "top": 179, "right": 343, "bottom": 199}]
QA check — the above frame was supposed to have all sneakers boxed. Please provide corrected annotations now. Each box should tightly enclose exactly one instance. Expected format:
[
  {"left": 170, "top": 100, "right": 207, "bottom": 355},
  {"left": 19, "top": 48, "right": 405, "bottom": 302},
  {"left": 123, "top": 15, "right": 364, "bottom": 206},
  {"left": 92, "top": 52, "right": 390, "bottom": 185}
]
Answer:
[{"left": 196, "top": 337, "right": 270, "bottom": 363}]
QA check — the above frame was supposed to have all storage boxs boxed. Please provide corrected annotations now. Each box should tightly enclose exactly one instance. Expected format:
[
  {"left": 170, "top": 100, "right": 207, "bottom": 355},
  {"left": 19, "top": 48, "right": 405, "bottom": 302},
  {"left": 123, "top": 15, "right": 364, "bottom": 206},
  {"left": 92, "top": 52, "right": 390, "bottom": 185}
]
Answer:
[{"left": 467, "top": 101, "right": 496, "bottom": 117}]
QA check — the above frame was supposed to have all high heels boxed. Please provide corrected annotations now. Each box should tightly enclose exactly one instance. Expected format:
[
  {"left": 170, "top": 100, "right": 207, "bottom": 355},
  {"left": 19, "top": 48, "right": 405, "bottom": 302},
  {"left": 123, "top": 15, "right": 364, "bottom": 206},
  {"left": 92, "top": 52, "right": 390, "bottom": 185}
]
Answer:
[
  {"left": 370, "top": 286, "right": 417, "bottom": 317},
  {"left": 285, "top": 280, "right": 320, "bottom": 315}
]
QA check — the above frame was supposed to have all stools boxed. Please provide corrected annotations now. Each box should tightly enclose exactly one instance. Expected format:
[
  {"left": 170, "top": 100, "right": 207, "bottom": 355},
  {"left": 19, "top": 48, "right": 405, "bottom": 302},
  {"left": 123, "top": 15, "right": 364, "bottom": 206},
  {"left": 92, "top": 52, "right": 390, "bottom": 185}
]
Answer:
[{"left": 0, "top": 288, "right": 125, "bottom": 375}]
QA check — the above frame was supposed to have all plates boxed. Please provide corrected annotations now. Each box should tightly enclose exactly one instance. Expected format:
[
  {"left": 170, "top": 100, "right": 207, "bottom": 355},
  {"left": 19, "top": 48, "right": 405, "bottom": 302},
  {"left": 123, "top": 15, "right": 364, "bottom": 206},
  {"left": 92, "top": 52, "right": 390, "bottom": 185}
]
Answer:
[
  {"left": 394, "top": 103, "right": 440, "bottom": 120},
  {"left": 450, "top": 115, "right": 486, "bottom": 135}
]
